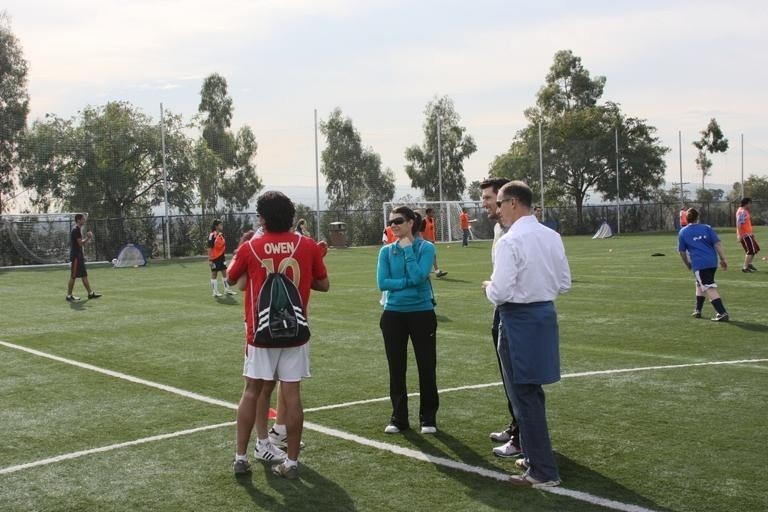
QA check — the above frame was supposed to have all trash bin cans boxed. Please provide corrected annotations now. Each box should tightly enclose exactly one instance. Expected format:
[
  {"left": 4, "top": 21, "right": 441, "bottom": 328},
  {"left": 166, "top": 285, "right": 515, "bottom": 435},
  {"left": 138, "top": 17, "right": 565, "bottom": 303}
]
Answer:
[{"left": 328, "top": 222, "right": 347, "bottom": 246}]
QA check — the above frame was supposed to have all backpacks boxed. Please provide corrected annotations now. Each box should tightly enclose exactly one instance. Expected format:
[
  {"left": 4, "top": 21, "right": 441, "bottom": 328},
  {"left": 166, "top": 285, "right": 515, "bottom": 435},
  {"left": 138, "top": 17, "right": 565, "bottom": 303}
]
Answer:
[{"left": 248, "top": 232, "right": 310, "bottom": 348}]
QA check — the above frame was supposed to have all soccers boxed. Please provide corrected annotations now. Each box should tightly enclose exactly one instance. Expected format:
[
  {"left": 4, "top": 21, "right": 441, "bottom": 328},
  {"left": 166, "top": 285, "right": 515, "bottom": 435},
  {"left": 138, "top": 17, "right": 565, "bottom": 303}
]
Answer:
[{"left": 608, "top": 248, "right": 614, "bottom": 253}]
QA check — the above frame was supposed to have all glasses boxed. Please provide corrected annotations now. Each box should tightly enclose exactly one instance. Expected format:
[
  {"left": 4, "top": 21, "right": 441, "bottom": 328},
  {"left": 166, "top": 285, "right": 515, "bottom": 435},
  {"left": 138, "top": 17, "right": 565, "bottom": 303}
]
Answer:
[
  {"left": 386, "top": 217, "right": 406, "bottom": 226},
  {"left": 495, "top": 198, "right": 519, "bottom": 208}
]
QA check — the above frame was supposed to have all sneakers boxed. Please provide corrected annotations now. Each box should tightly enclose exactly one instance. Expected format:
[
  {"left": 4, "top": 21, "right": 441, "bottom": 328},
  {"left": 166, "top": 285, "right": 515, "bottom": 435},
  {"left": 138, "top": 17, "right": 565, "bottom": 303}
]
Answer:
[
  {"left": 66, "top": 295, "right": 80, "bottom": 300},
  {"left": 212, "top": 291, "right": 222, "bottom": 297},
  {"left": 88, "top": 291, "right": 102, "bottom": 299},
  {"left": 691, "top": 310, "right": 701, "bottom": 317},
  {"left": 489, "top": 430, "right": 561, "bottom": 488},
  {"left": 233, "top": 428, "right": 304, "bottom": 480},
  {"left": 710, "top": 313, "right": 727, "bottom": 321},
  {"left": 223, "top": 289, "right": 237, "bottom": 295},
  {"left": 420, "top": 426, "right": 436, "bottom": 434},
  {"left": 435, "top": 271, "right": 447, "bottom": 277},
  {"left": 741, "top": 268, "right": 754, "bottom": 272},
  {"left": 384, "top": 424, "right": 400, "bottom": 434}
]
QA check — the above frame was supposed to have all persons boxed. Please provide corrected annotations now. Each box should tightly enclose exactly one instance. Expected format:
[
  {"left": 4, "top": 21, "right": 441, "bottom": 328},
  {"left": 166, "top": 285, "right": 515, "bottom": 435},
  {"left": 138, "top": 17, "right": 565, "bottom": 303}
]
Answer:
[
  {"left": 676, "top": 207, "right": 730, "bottom": 322},
  {"left": 235, "top": 223, "right": 329, "bottom": 463},
  {"left": 376, "top": 205, "right": 440, "bottom": 434},
  {"left": 379, "top": 205, "right": 400, "bottom": 308},
  {"left": 735, "top": 197, "right": 761, "bottom": 273},
  {"left": 459, "top": 206, "right": 471, "bottom": 248},
  {"left": 480, "top": 177, "right": 572, "bottom": 488},
  {"left": 65, "top": 213, "right": 103, "bottom": 301},
  {"left": 205, "top": 218, "right": 239, "bottom": 298},
  {"left": 480, "top": 176, "right": 523, "bottom": 458},
  {"left": 532, "top": 206, "right": 542, "bottom": 222},
  {"left": 292, "top": 217, "right": 311, "bottom": 239},
  {"left": 224, "top": 188, "right": 330, "bottom": 479},
  {"left": 679, "top": 206, "right": 688, "bottom": 227},
  {"left": 417, "top": 208, "right": 448, "bottom": 278}
]
